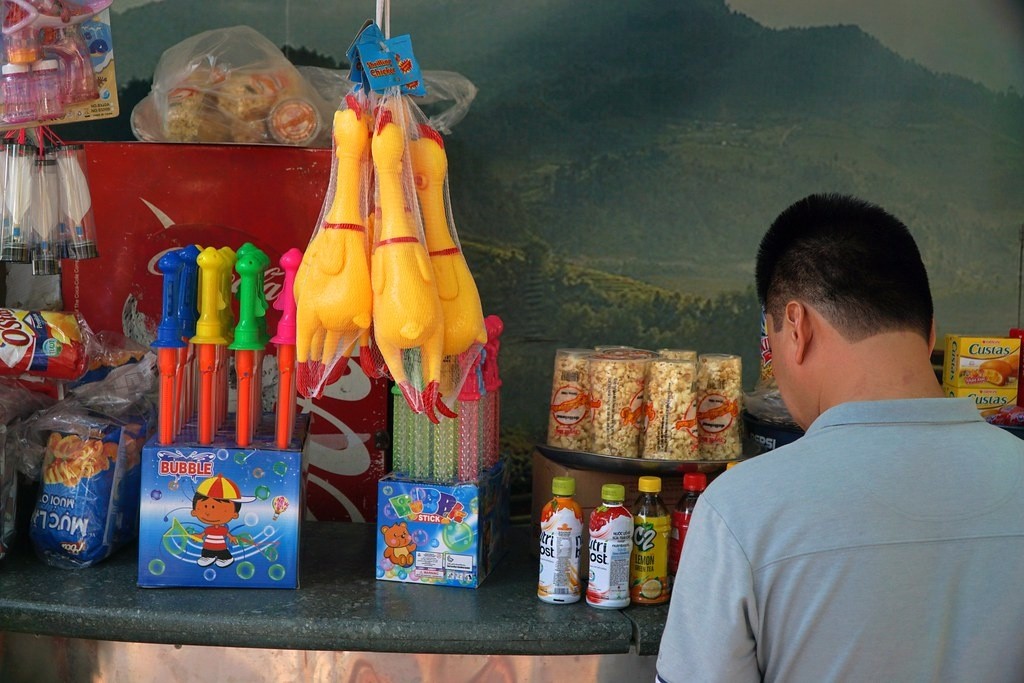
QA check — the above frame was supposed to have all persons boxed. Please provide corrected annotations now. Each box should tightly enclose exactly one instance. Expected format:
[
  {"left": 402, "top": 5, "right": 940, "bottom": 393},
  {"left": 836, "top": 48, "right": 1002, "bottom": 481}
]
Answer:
[{"left": 656, "top": 194, "right": 1024, "bottom": 683}]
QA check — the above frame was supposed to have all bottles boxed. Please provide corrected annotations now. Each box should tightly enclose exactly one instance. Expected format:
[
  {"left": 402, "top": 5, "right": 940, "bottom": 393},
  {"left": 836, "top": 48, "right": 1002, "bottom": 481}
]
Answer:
[
  {"left": 537, "top": 477, "right": 583, "bottom": 604},
  {"left": 2, "top": 63, "right": 34, "bottom": 122},
  {"left": 632, "top": 477, "right": 671, "bottom": 604},
  {"left": 587, "top": 483, "right": 633, "bottom": 609},
  {"left": 672, "top": 472, "right": 707, "bottom": 581},
  {"left": 32, "top": 59, "right": 64, "bottom": 119}
]
